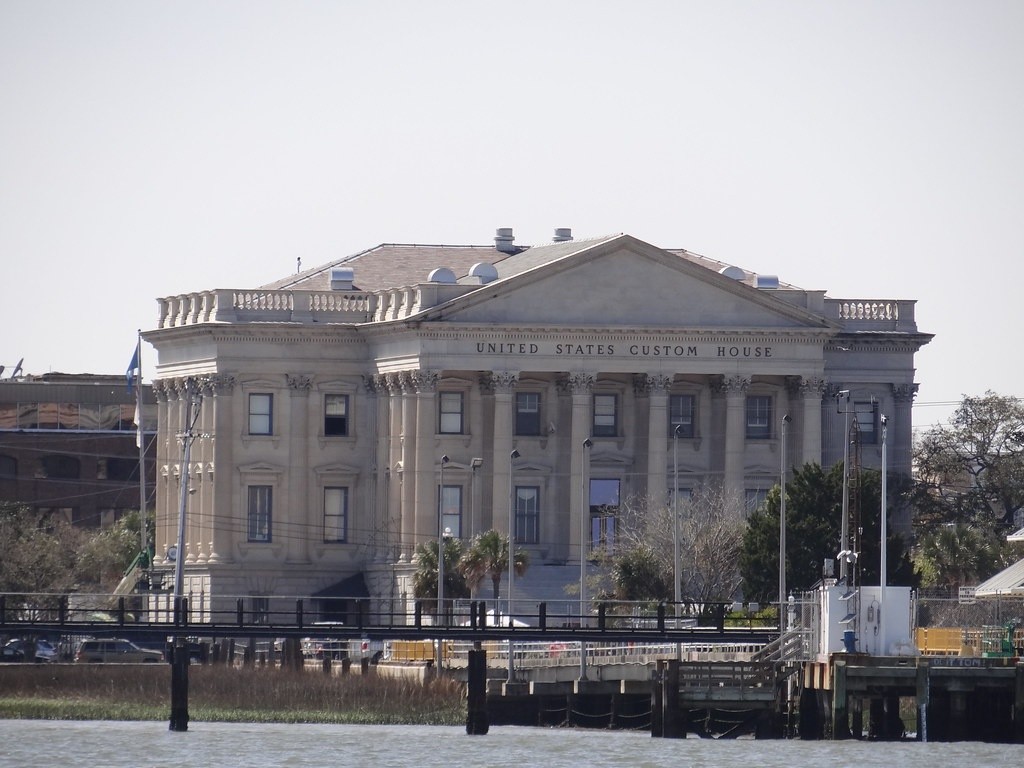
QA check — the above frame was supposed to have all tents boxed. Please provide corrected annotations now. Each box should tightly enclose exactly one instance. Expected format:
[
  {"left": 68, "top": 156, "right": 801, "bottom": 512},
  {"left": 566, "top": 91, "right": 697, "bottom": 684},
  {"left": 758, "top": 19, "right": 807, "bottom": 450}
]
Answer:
[{"left": 460, "top": 608, "right": 530, "bottom": 658}]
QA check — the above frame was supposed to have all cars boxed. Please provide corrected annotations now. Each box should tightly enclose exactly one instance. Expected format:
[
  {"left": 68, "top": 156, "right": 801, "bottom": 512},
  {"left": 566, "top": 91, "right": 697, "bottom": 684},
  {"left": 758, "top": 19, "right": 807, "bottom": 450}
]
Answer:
[
  {"left": 277, "top": 622, "right": 390, "bottom": 667},
  {"left": 5, "top": 639, "right": 57, "bottom": 660}
]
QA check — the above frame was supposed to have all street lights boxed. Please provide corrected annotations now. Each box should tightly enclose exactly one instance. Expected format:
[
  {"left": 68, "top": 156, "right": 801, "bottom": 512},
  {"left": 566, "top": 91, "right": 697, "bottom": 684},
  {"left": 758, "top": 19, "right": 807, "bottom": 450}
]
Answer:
[
  {"left": 510, "top": 449, "right": 520, "bottom": 623},
  {"left": 780, "top": 415, "right": 791, "bottom": 601},
  {"left": 673, "top": 425, "right": 684, "bottom": 630},
  {"left": 580, "top": 438, "right": 592, "bottom": 627},
  {"left": 438, "top": 455, "right": 452, "bottom": 624}
]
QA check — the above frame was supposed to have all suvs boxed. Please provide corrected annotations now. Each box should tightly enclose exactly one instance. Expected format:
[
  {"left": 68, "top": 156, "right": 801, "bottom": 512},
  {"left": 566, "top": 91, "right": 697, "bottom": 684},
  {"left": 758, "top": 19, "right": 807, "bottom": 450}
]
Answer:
[{"left": 74, "top": 637, "right": 164, "bottom": 664}]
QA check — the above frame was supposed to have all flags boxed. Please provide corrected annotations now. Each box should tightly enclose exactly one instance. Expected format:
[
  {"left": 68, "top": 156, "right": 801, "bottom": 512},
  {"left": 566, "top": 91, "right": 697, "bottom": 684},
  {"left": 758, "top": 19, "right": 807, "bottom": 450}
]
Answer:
[
  {"left": 126, "top": 347, "right": 138, "bottom": 394},
  {"left": 134, "top": 391, "right": 141, "bottom": 447}
]
